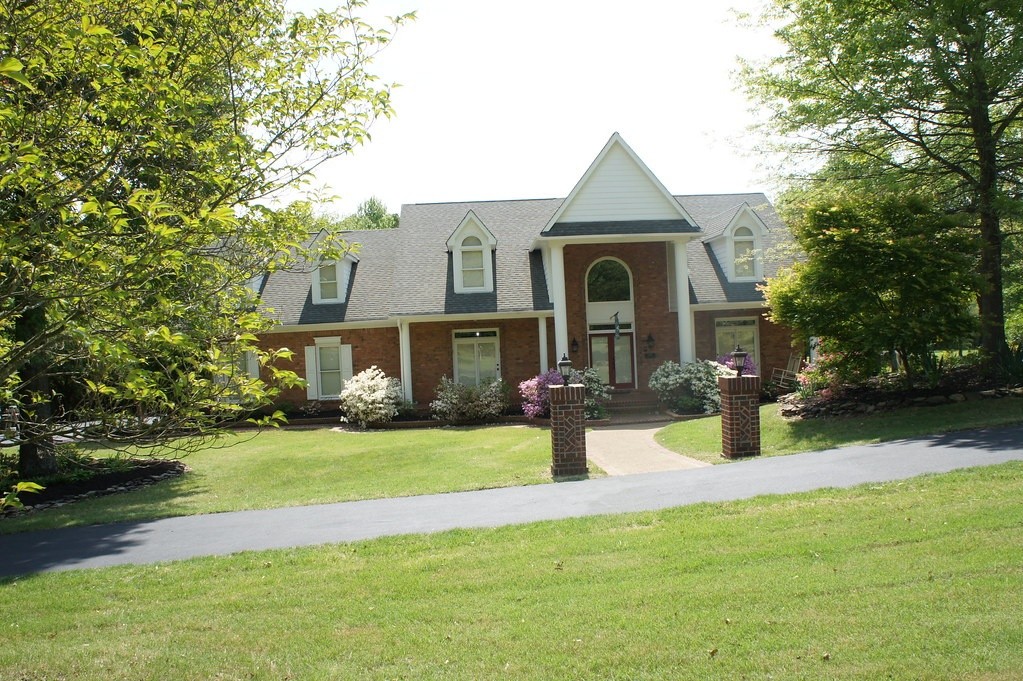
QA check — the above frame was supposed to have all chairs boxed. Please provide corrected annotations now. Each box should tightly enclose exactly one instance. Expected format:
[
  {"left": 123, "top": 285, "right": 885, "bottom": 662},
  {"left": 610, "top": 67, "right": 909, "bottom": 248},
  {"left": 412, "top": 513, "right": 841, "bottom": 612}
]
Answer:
[{"left": 771, "top": 351, "right": 803, "bottom": 389}]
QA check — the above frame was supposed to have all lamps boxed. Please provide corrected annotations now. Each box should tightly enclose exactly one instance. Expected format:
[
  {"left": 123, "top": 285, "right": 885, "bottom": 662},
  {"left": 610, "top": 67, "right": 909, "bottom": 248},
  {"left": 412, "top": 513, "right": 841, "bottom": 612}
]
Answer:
[
  {"left": 730, "top": 345, "right": 748, "bottom": 377},
  {"left": 644, "top": 333, "right": 656, "bottom": 359},
  {"left": 571, "top": 336, "right": 579, "bottom": 352},
  {"left": 558, "top": 353, "right": 573, "bottom": 387}
]
